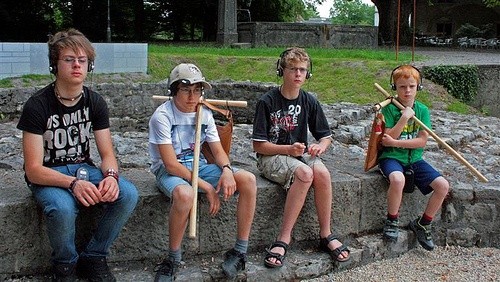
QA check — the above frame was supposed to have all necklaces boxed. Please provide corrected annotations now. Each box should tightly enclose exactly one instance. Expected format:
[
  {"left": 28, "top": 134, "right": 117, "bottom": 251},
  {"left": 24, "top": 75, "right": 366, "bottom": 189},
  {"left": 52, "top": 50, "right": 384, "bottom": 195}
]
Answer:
[{"left": 52, "top": 83, "right": 84, "bottom": 102}]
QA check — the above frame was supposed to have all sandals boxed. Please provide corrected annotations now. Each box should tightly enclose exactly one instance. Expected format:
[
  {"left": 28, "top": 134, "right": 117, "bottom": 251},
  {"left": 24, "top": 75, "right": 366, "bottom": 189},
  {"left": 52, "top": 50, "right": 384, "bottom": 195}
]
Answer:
[
  {"left": 320, "top": 233, "right": 351, "bottom": 262},
  {"left": 264, "top": 238, "right": 289, "bottom": 267}
]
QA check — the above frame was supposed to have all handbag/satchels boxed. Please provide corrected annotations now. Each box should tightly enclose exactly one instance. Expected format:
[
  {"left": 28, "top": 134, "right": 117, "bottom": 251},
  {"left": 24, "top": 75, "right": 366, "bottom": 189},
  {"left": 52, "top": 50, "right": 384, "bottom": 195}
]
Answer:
[
  {"left": 364, "top": 113, "right": 384, "bottom": 173},
  {"left": 199, "top": 100, "right": 233, "bottom": 164}
]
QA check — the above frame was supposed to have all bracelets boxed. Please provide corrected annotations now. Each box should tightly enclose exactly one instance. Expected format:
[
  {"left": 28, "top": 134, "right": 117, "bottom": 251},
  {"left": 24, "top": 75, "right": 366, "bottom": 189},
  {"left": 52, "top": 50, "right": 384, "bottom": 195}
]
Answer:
[
  {"left": 103, "top": 168, "right": 119, "bottom": 183},
  {"left": 69, "top": 178, "right": 79, "bottom": 193}
]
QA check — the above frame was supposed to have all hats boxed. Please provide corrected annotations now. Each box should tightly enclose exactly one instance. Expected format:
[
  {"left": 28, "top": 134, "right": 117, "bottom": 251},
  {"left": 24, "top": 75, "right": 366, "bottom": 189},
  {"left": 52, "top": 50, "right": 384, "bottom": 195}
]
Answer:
[{"left": 169, "top": 63, "right": 212, "bottom": 89}]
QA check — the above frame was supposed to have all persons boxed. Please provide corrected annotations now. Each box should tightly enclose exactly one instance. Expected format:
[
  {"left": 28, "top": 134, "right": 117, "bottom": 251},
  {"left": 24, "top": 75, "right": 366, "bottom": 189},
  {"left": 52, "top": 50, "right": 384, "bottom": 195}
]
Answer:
[
  {"left": 16, "top": 28, "right": 138, "bottom": 282},
  {"left": 376, "top": 65, "right": 449, "bottom": 250},
  {"left": 148, "top": 63, "right": 257, "bottom": 282},
  {"left": 252, "top": 48, "right": 351, "bottom": 267}
]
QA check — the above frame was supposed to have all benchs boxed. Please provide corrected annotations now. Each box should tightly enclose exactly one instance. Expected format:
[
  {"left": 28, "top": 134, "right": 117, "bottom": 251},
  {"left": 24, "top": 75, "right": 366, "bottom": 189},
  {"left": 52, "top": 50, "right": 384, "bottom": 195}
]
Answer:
[{"left": 380, "top": 36, "right": 393, "bottom": 47}]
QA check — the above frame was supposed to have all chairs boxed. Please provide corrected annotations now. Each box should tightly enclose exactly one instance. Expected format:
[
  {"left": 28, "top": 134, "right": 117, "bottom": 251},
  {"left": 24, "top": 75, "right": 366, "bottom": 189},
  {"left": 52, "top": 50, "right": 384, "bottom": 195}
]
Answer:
[{"left": 415, "top": 35, "right": 500, "bottom": 51}]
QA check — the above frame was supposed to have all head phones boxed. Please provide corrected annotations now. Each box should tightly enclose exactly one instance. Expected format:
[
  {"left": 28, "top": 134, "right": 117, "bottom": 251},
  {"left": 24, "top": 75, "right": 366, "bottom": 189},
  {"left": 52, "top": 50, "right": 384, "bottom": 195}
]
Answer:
[
  {"left": 167, "top": 69, "right": 204, "bottom": 97},
  {"left": 277, "top": 49, "right": 312, "bottom": 79},
  {"left": 390, "top": 65, "right": 423, "bottom": 91},
  {"left": 48, "top": 35, "right": 94, "bottom": 74}
]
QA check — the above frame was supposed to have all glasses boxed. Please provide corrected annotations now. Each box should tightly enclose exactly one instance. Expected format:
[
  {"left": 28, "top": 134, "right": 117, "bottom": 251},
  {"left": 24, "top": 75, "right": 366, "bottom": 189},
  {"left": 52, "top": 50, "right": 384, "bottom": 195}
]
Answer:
[
  {"left": 286, "top": 67, "right": 308, "bottom": 74},
  {"left": 59, "top": 57, "right": 87, "bottom": 64},
  {"left": 179, "top": 88, "right": 202, "bottom": 94}
]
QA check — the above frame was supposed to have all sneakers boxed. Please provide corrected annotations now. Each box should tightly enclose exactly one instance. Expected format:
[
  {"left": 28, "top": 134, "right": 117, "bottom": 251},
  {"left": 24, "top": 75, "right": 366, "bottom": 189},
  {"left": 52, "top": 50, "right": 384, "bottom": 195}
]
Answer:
[
  {"left": 52, "top": 262, "right": 79, "bottom": 282},
  {"left": 152, "top": 258, "right": 179, "bottom": 282},
  {"left": 409, "top": 217, "right": 434, "bottom": 251},
  {"left": 383, "top": 218, "right": 400, "bottom": 240},
  {"left": 84, "top": 256, "right": 116, "bottom": 282},
  {"left": 222, "top": 248, "right": 246, "bottom": 278}
]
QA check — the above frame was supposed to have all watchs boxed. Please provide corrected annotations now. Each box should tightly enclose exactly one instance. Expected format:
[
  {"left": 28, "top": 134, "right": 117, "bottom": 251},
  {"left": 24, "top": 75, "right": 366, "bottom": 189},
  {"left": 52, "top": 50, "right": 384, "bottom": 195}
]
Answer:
[{"left": 222, "top": 163, "right": 232, "bottom": 171}]
